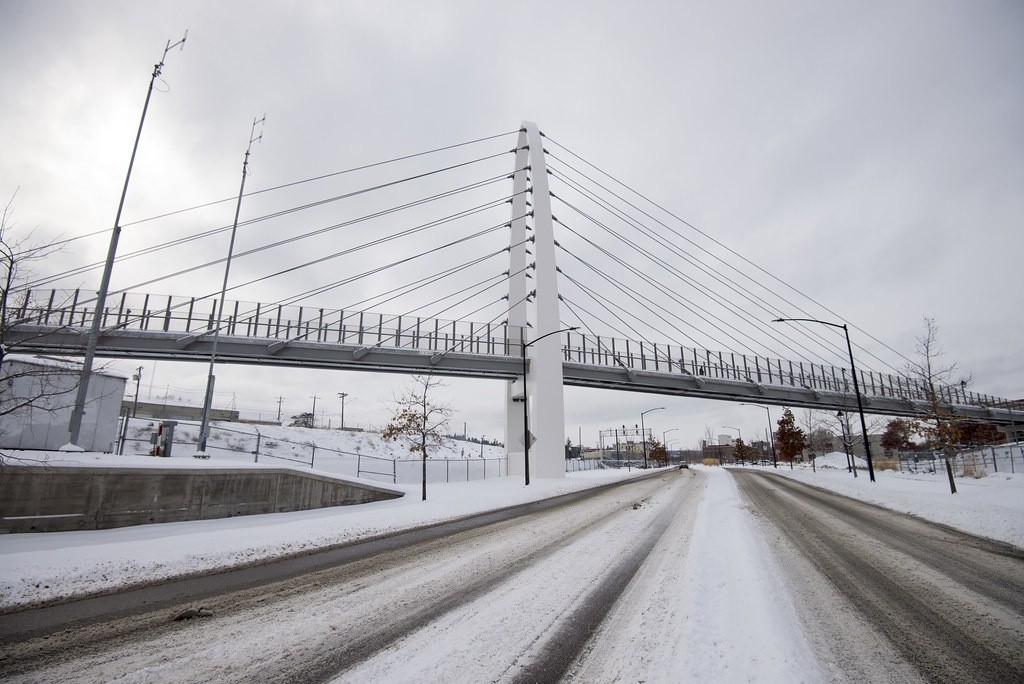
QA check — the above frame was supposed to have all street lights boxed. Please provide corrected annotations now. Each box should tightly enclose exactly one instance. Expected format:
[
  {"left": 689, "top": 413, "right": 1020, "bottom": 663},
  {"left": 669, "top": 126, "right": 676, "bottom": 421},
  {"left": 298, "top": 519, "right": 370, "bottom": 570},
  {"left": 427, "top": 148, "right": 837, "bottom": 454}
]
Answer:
[
  {"left": 771, "top": 317, "right": 876, "bottom": 484},
  {"left": 663, "top": 428, "right": 679, "bottom": 468},
  {"left": 722, "top": 426, "right": 744, "bottom": 466},
  {"left": 739, "top": 403, "right": 777, "bottom": 468},
  {"left": 519, "top": 325, "right": 582, "bottom": 485},
  {"left": 836, "top": 410, "right": 852, "bottom": 472},
  {"left": 666, "top": 438, "right": 680, "bottom": 465},
  {"left": 641, "top": 407, "right": 666, "bottom": 468},
  {"left": 707, "top": 438, "right": 722, "bottom": 466}
]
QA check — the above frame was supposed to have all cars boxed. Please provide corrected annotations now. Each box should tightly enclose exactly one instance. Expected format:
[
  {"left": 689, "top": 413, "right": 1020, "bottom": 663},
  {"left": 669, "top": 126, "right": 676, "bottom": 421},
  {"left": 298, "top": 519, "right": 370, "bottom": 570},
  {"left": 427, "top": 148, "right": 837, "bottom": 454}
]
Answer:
[{"left": 678, "top": 461, "right": 689, "bottom": 469}]
